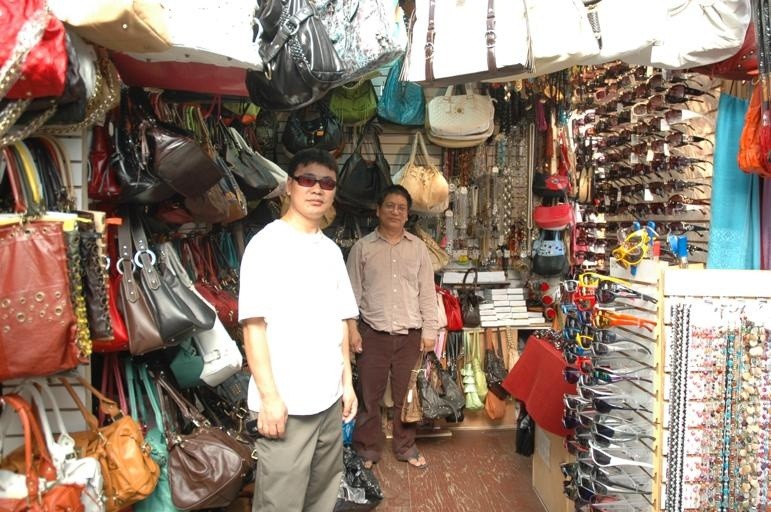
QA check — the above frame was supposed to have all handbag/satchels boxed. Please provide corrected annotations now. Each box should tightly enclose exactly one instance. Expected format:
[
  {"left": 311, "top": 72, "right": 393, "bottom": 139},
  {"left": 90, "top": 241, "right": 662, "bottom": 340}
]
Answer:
[
  {"left": 530, "top": 171, "right": 571, "bottom": 276},
  {"left": 394, "top": 134, "right": 448, "bottom": 273},
  {"left": 424, "top": 87, "right": 497, "bottom": 148},
  {"left": 516, "top": 411, "right": 536, "bottom": 456},
  {"left": 400, "top": 269, "right": 510, "bottom": 424}
]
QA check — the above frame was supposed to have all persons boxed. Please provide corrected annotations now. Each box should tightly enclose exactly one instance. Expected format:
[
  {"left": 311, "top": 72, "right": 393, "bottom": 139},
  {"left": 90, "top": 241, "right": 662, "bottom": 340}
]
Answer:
[
  {"left": 238, "top": 145, "right": 361, "bottom": 512},
  {"left": 347, "top": 184, "right": 441, "bottom": 469}
]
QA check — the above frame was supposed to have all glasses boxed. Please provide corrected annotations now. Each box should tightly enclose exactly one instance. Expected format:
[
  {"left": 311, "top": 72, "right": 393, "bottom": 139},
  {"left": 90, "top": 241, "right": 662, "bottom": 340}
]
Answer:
[
  {"left": 574, "top": 67, "right": 716, "bottom": 263},
  {"left": 558, "top": 272, "right": 658, "bottom": 512}
]
